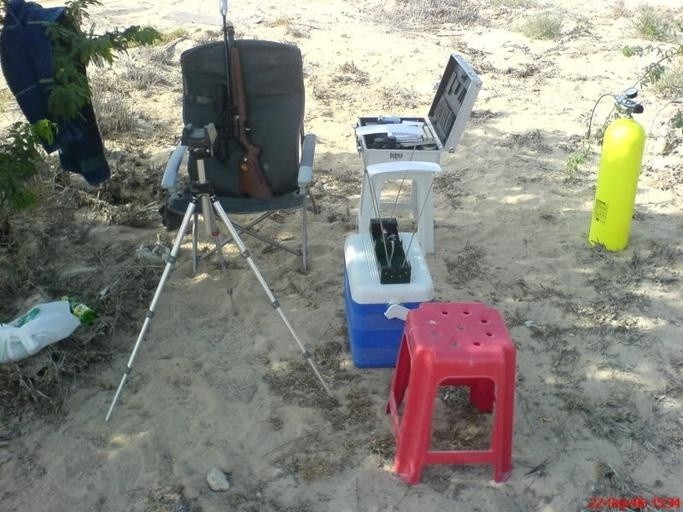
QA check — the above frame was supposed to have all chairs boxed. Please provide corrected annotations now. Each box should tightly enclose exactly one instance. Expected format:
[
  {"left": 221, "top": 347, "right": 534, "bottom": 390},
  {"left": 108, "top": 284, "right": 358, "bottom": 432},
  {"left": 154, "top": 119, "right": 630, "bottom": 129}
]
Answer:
[{"left": 160, "top": 40, "right": 318, "bottom": 273}]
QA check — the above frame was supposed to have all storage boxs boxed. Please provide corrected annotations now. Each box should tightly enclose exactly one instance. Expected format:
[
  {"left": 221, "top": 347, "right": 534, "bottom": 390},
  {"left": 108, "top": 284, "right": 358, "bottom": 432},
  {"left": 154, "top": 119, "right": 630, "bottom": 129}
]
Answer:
[
  {"left": 343, "top": 231, "right": 435, "bottom": 369},
  {"left": 353, "top": 54, "right": 483, "bottom": 167}
]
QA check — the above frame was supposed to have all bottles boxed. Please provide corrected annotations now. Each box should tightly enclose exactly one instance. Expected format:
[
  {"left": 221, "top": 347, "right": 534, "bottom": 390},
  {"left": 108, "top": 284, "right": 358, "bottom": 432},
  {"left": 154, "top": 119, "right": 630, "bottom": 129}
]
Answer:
[{"left": 62, "top": 296, "right": 97, "bottom": 323}]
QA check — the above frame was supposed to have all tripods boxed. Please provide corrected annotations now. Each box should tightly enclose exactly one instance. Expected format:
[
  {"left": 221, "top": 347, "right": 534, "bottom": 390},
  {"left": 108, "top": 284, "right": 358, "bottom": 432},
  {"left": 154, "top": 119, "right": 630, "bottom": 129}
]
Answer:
[{"left": 104, "top": 122, "right": 339, "bottom": 423}]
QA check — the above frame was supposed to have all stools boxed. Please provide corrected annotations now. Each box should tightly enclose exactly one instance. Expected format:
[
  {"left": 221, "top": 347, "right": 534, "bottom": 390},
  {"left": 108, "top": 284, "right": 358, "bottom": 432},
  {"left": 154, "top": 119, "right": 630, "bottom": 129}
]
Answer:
[
  {"left": 358, "top": 170, "right": 435, "bottom": 255},
  {"left": 384, "top": 303, "right": 517, "bottom": 485}
]
